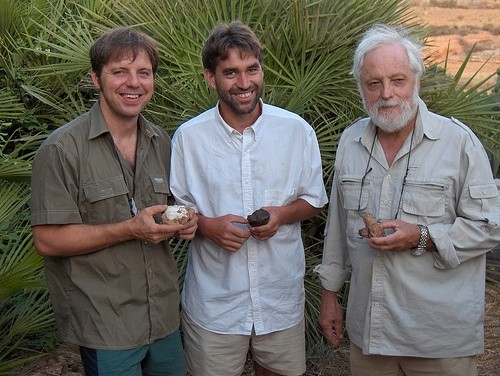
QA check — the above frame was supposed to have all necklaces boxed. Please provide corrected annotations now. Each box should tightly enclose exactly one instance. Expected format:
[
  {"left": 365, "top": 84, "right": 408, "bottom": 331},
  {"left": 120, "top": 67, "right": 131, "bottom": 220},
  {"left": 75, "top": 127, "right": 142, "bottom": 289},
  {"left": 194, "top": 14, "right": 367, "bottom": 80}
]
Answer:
[{"left": 113, "top": 120, "right": 140, "bottom": 217}]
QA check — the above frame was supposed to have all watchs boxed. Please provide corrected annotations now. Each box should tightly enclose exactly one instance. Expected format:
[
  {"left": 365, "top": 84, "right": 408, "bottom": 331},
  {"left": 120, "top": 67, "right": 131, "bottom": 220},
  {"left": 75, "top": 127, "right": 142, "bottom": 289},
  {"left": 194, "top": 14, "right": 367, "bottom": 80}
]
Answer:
[{"left": 410, "top": 224, "right": 428, "bottom": 257}]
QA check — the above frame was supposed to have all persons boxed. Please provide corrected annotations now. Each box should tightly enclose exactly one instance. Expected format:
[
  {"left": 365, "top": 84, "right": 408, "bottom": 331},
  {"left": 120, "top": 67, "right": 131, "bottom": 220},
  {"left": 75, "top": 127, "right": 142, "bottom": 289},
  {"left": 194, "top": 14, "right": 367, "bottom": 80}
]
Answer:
[
  {"left": 30, "top": 27, "right": 198, "bottom": 376},
  {"left": 313, "top": 23, "right": 500, "bottom": 376},
  {"left": 169, "top": 21, "right": 328, "bottom": 376}
]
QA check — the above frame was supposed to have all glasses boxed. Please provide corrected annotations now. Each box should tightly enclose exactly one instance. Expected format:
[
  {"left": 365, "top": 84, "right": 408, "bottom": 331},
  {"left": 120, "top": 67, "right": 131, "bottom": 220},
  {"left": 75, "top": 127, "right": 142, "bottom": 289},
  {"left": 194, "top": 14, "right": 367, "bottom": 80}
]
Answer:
[{"left": 358, "top": 168, "right": 406, "bottom": 222}]
